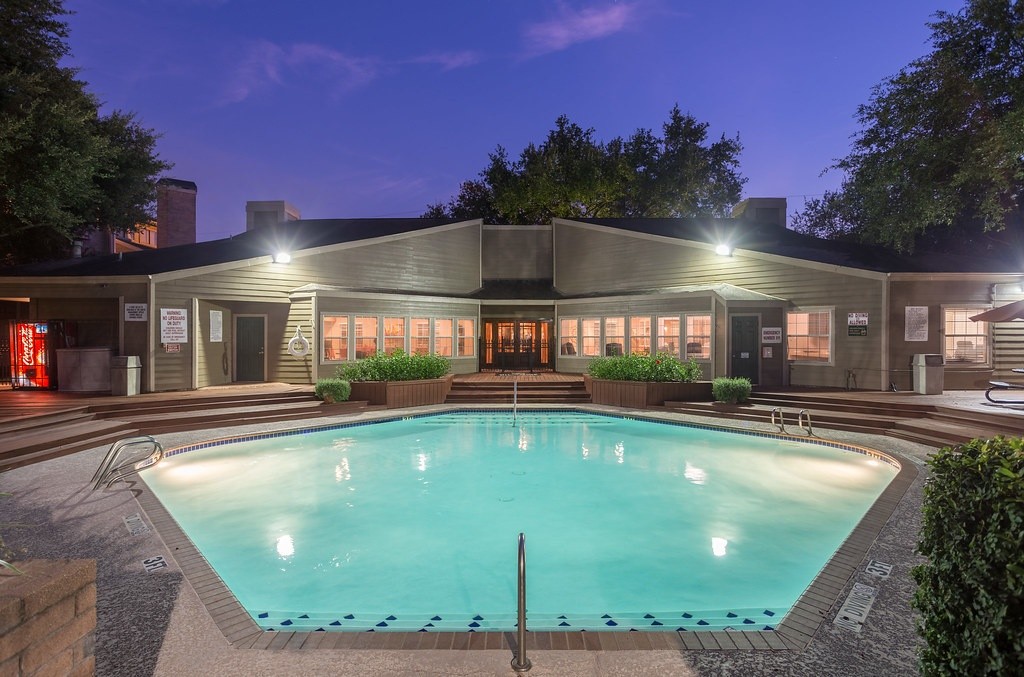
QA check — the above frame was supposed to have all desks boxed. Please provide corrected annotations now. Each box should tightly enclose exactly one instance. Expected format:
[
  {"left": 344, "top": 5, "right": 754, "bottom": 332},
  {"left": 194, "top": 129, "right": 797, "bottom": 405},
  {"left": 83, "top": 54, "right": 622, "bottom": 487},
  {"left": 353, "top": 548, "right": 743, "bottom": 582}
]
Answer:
[{"left": 790, "top": 351, "right": 828, "bottom": 362}]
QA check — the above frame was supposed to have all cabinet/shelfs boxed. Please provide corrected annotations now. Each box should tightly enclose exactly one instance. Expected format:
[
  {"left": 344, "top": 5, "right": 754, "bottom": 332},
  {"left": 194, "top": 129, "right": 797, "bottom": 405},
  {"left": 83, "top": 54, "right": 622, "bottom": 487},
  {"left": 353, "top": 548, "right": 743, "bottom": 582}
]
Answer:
[{"left": 55, "top": 348, "right": 111, "bottom": 392}]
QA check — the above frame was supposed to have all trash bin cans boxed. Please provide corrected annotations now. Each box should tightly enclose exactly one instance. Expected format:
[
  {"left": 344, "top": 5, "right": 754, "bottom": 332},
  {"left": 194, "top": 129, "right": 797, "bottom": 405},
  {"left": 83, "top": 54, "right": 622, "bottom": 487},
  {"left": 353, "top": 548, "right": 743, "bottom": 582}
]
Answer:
[
  {"left": 911, "top": 353, "right": 946, "bottom": 395},
  {"left": 109, "top": 355, "right": 143, "bottom": 396}
]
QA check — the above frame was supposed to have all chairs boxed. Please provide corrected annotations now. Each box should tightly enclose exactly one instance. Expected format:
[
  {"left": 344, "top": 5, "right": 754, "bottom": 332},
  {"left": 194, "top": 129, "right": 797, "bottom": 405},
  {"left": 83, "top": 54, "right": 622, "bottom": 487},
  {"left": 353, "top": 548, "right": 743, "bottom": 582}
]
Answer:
[
  {"left": 687, "top": 343, "right": 704, "bottom": 357},
  {"left": 566, "top": 342, "right": 577, "bottom": 355},
  {"left": 606, "top": 343, "right": 624, "bottom": 356}
]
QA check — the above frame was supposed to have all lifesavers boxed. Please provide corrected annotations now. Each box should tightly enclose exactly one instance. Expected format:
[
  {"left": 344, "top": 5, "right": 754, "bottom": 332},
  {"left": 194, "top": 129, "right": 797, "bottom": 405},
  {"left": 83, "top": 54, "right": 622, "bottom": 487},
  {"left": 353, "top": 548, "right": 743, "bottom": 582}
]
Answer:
[{"left": 289, "top": 338, "right": 310, "bottom": 358}]
